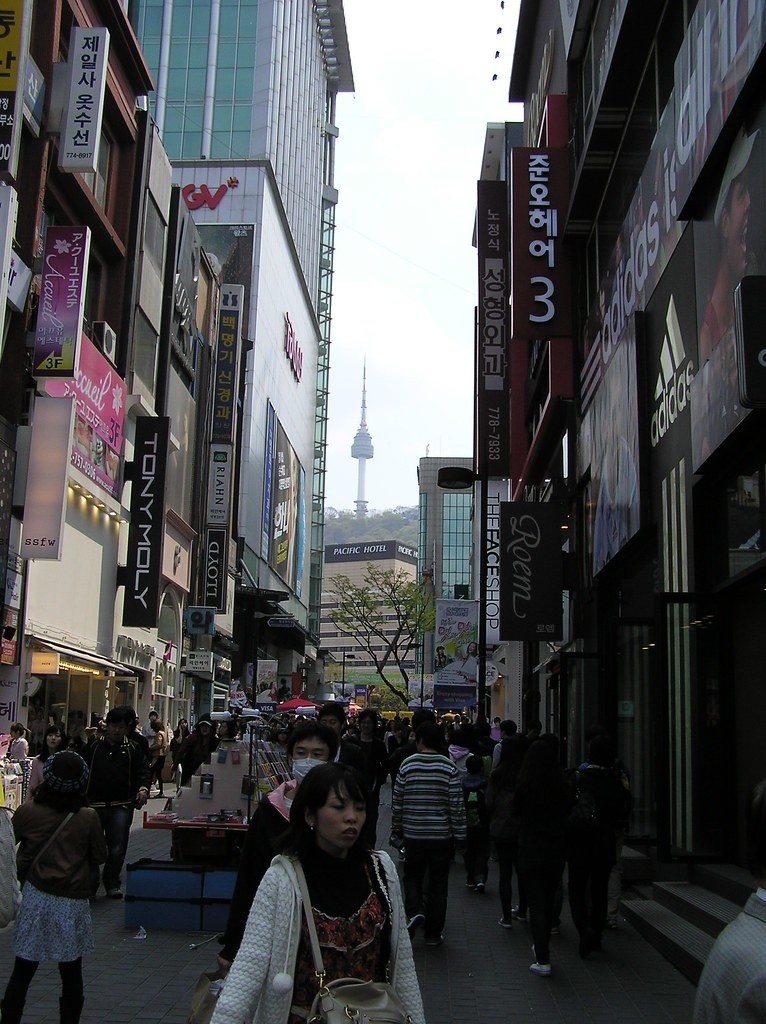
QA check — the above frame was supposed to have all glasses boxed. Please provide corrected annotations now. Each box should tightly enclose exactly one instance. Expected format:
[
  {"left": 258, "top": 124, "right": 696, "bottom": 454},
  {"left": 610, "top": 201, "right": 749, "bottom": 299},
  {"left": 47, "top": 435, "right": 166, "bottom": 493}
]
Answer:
[{"left": 63, "top": 743, "right": 78, "bottom": 748}]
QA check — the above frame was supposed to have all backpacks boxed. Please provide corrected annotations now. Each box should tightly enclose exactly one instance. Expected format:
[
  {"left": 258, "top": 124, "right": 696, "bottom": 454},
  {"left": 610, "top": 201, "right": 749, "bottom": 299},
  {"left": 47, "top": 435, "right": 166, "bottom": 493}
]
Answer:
[{"left": 461, "top": 776, "right": 490, "bottom": 837}]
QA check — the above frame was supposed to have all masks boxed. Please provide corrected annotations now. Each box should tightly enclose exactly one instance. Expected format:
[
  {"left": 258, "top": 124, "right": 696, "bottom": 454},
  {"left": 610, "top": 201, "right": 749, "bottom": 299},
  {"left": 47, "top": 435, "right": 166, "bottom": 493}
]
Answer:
[{"left": 292, "top": 758, "right": 328, "bottom": 785}]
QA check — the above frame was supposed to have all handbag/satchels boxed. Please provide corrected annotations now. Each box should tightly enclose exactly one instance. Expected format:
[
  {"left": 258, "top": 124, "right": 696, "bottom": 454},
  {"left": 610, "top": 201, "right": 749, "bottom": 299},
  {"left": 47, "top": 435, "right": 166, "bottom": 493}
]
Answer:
[
  {"left": 565, "top": 767, "right": 602, "bottom": 826},
  {"left": 307, "top": 978, "right": 413, "bottom": 1024},
  {"left": 188, "top": 967, "right": 228, "bottom": 1024}
]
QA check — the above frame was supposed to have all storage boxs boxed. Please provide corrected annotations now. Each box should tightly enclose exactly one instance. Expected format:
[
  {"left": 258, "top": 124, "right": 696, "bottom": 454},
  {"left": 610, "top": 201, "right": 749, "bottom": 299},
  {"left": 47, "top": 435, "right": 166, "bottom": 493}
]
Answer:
[{"left": 123, "top": 857, "right": 239, "bottom": 932}]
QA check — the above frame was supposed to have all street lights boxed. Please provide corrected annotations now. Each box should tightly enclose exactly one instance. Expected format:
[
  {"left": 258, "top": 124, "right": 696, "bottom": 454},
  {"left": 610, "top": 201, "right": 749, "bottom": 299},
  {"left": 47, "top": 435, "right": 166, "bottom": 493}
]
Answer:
[
  {"left": 409, "top": 634, "right": 425, "bottom": 710},
  {"left": 342, "top": 646, "right": 356, "bottom": 696},
  {"left": 436, "top": 466, "right": 488, "bottom": 723}
]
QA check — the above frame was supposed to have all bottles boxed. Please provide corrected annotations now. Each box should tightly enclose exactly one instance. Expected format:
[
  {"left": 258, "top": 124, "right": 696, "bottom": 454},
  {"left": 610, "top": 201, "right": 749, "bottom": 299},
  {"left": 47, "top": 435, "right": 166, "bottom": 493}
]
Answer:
[{"left": 389, "top": 834, "right": 406, "bottom": 854}]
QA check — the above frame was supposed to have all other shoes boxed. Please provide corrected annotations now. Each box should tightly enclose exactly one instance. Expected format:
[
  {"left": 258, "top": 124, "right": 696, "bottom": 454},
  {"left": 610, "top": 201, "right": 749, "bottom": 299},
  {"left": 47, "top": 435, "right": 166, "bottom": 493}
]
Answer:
[
  {"left": 464, "top": 879, "right": 485, "bottom": 896},
  {"left": 511, "top": 905, "right": 526, "bottom": 921},
  {"left": 578, "top": 925, "right": 592, "bottom": 960},
  {"left": 550, "top": 924, "right": 561, "bottom": 935},
  {"left": 498, "top": 918, "right": 513, "bottom": 928},
  {"left": 424, "top": 934, "right": 443, "bottom": 946},
  {"left": 605, "top": 915, "right": 617, "bottom": 929},
  {"left": 89, "top": 887, "right": 98, "bottom": 901},
  {"left": 407, "top": 912, "right": 426, "bottom": 940},
  {"left": 107, "top": 887, "right": 123, "bottom": 899},
  {"left": 588, "top": 933, "right": 602, "bottom": 951}
]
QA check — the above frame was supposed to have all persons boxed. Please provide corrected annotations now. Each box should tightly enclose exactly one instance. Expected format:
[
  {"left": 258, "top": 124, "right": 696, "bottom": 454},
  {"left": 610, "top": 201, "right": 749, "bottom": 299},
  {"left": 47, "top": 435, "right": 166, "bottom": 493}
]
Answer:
[
  {"left": 593, "top": 347, "right": 635, "bottom": 575},
  {"left": 698, "top": 125, "right": 761, "bottom": 366},
  {"left": 458, "top": 641, "right": 477, "bottom": 682},
  {"left": 435, "top": 646, "right": 447, "bottom": 672},
  {"left": 693, "top": 779, "right": 766, "bottom": 1024},
  {"left": 216, "top": 720, "right": 337, "bottom": 970},
  {"left": 208, "top": 760, "right": 425, "bottom": 1024},
  {"left": 0, "top": 749, "right": 109, "bottom": 1024},
  {"left": 0, "top": 702, "right": 635, "bottom": 977}
]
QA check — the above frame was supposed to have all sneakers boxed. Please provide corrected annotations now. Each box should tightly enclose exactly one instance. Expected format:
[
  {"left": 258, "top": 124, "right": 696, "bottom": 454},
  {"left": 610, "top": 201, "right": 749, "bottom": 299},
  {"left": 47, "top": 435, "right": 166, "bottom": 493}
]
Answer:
[
  {"left": 529, "top": 962, "right": 552, "bottom": 977},
  {"left": 530, "top": 943, "right": 538, "bottom": 963}
]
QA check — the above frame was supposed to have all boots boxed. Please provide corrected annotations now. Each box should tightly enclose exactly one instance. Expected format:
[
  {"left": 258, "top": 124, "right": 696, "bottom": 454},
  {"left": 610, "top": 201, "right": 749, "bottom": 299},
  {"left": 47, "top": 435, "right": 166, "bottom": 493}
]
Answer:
[
  {"left": 59, "top": 996, "right": 84, "bottom": 1024},
  {"left": 0, "top": 999, "right": 26, "bottom": 1024}
]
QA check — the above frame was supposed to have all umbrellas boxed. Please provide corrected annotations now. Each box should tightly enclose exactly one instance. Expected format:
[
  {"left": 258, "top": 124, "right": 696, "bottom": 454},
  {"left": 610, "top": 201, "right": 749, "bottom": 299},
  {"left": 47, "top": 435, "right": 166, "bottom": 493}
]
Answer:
[{"left": 276, "top": 698, "right": 323, "bottom": 712}]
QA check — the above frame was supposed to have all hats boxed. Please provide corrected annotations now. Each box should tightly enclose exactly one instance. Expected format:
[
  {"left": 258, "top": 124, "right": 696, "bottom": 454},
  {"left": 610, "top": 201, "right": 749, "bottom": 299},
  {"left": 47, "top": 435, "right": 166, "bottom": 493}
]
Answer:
[
  {"left": 198, "top": 721, "right": 213, "bottom": 727},
  {"left": 43, "top": 750, "right": 90, "bottom": 795}
]
